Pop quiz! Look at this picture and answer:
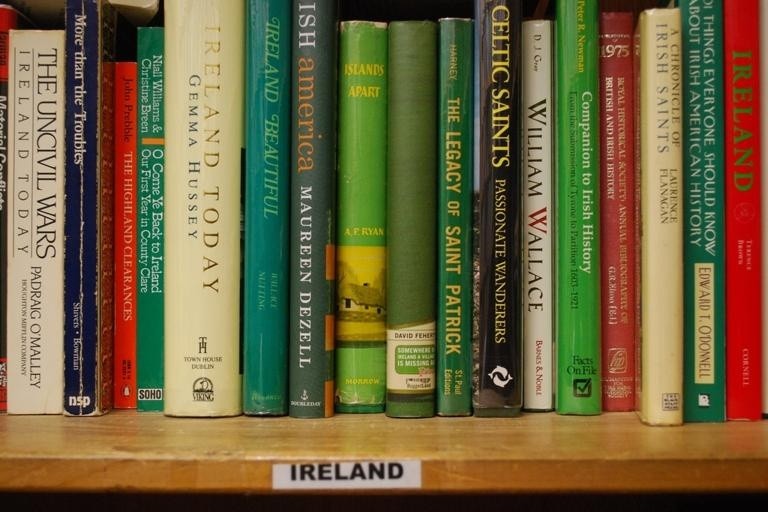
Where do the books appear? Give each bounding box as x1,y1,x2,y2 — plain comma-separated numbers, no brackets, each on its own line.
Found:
136,26,165,413
601,9,635,414
520,19,555,414
163,1,241,420
724,1,766,423
680,1,725,427
387,20,436,419
62,0,110,417
472,1,521,417
552,1,601,417
437,16,471,418
6,28,64,417
335,19,387,416
640,7,684,427
110,59,137,413
290,0,335,419
243,1,290,417
